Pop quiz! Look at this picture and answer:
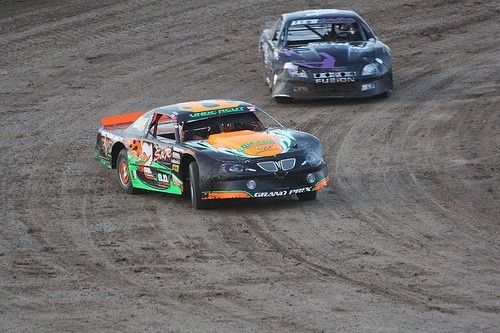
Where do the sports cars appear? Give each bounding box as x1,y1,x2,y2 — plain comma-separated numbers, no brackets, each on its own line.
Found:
94,99,329,210
259,9,393,103
